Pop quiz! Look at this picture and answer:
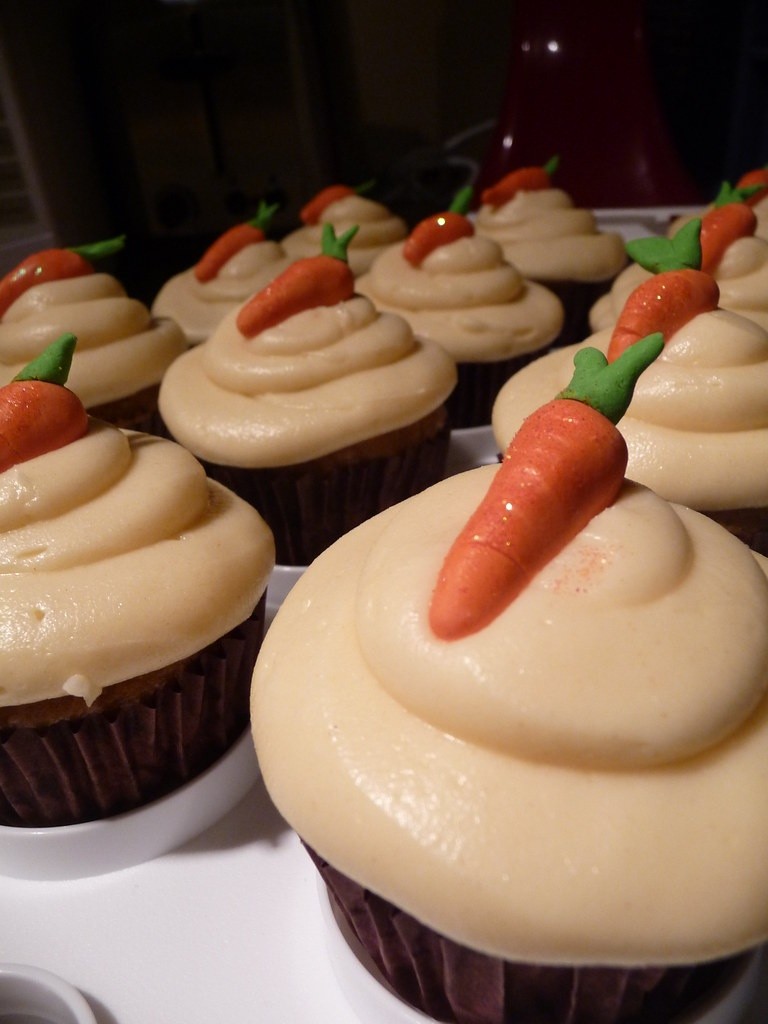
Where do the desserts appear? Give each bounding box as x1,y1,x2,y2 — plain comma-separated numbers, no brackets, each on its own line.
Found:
2,171,767,1024
